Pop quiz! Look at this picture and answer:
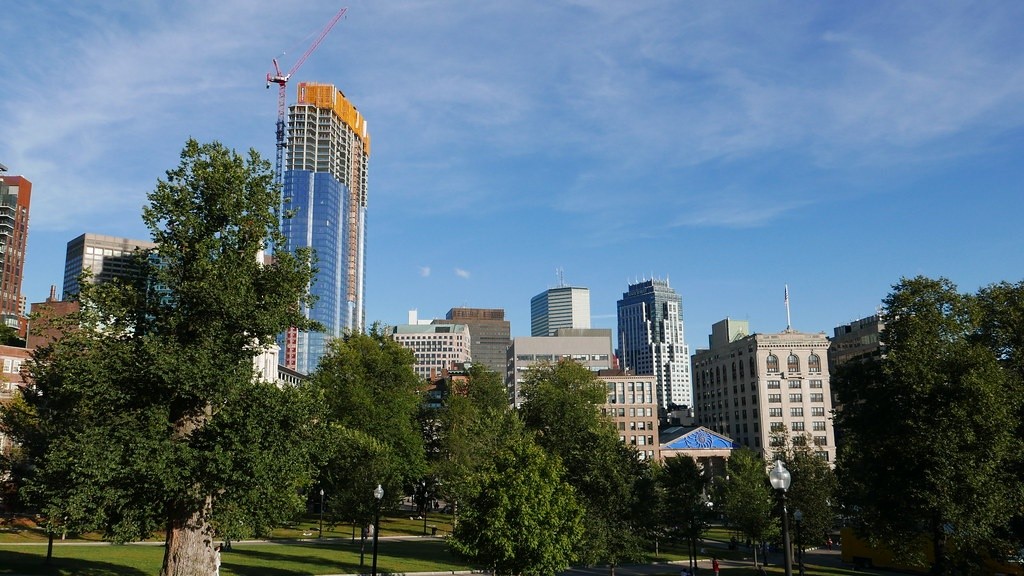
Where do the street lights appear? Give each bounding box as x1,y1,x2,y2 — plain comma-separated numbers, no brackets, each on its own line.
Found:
372,485,384,575
424,492,428,534
264,6,348,259
768,460,793,576
794,509,805,576
319,490,324,534
